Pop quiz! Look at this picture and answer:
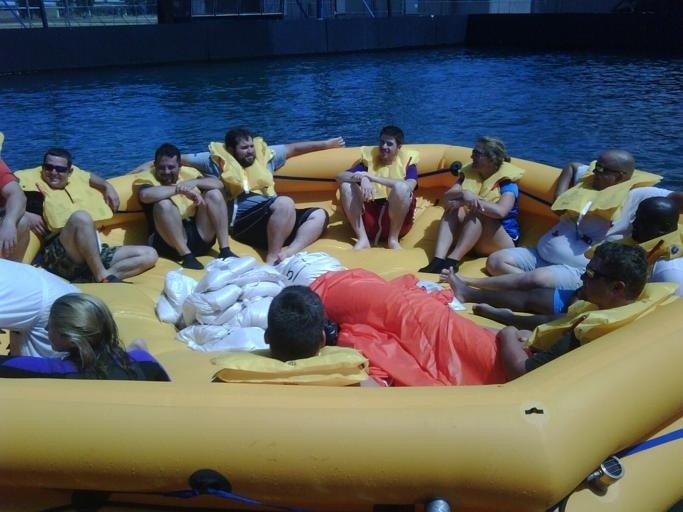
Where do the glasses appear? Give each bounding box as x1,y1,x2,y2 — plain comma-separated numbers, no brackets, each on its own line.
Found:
42,163,70,172
473,149,487,156
595,161,621,174
586,266,607,282
